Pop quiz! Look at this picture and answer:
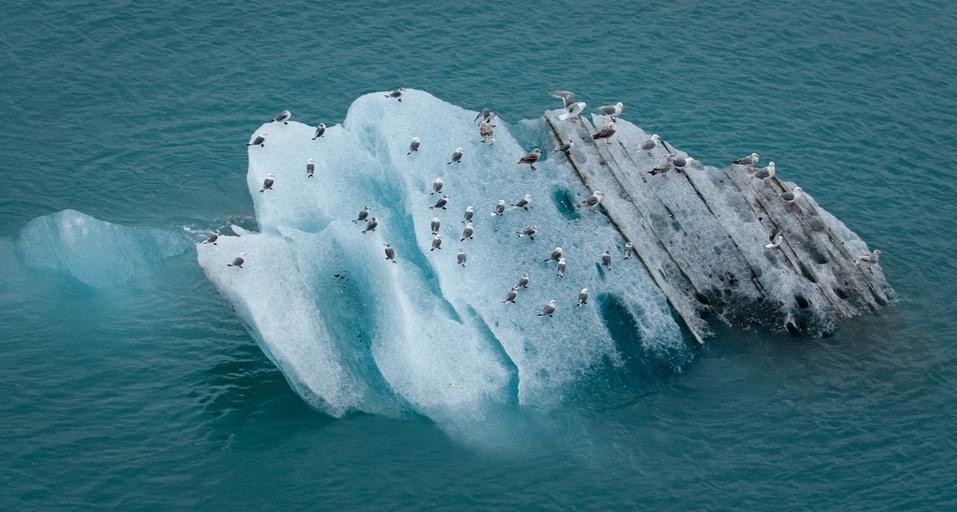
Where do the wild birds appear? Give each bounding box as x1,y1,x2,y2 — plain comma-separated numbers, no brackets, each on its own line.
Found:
749,162,776,189
247,109,326,195
516,224,538,242
591,102,624,123
429,177,451,252
225,255,246,270
640,133,696,180
552,139,575,157
577,287,589,308
446,148,464,167
624,240,634,261
516,148,543,171
853,250,882,275
765,222,785,250
547,90,586,123
474,109,497,144
381,243,398,264
455,204,478,269
204,229,220,246
538,299,559,318
384,87,406,103
592,121,619,145
352,205,379,235
500,272,529,306
492,199,506,218
775,186,802,206
544,246,567,280
733,152,761,173
602,250,613,272
577,189,605,217
510,194,532,211
408,137,422,156
